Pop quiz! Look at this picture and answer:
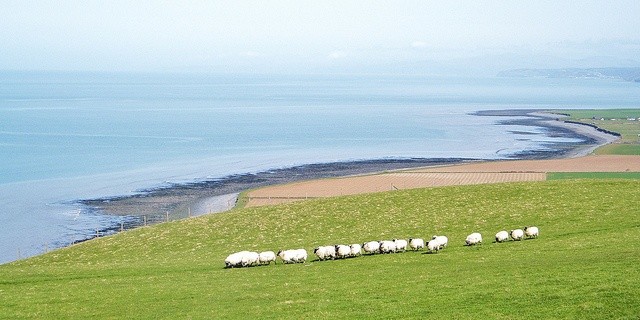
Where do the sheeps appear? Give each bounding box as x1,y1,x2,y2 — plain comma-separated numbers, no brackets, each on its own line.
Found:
524,226,539,239
277,249,298,265
297,248,307,263
225,251,250,268
241,252,259,267
314,246,336,261
335,244,351,259
259,251,276,266
380,240,396,254
377,240,388,254
362,241,380,255
510,229,524,241
495,230,508,242
433,235,448,248
465,233,482,246
408,239,424,251
393,239,408,253
349,244,362,257
426,239,441,253
